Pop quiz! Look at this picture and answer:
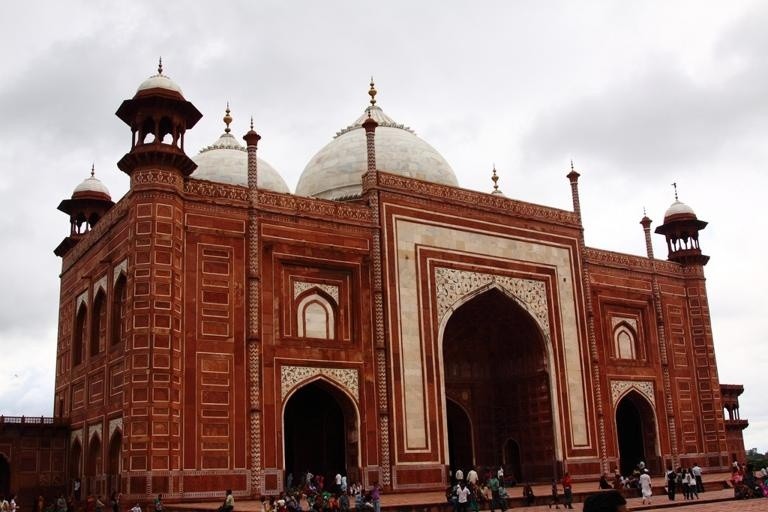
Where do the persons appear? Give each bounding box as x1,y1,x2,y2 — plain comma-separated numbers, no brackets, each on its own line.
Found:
111,491,119,512
665,462,705,501
599,457,653,505
96,494,106,512
549,472,574,509
86,491,97,512
445,464,515,512
73,476,80,501
127,501,142,512
57,492,69,512
259,467,382,512
523,481,534,506
152,492,165,511
730,458,767,500
0,490,20,512
218,488,234,511
